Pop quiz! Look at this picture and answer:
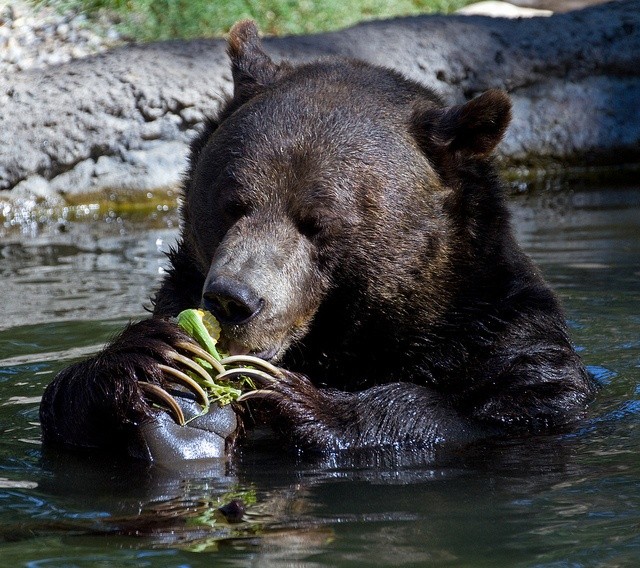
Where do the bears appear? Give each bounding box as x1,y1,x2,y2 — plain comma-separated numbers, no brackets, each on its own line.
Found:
38,19,596,464
